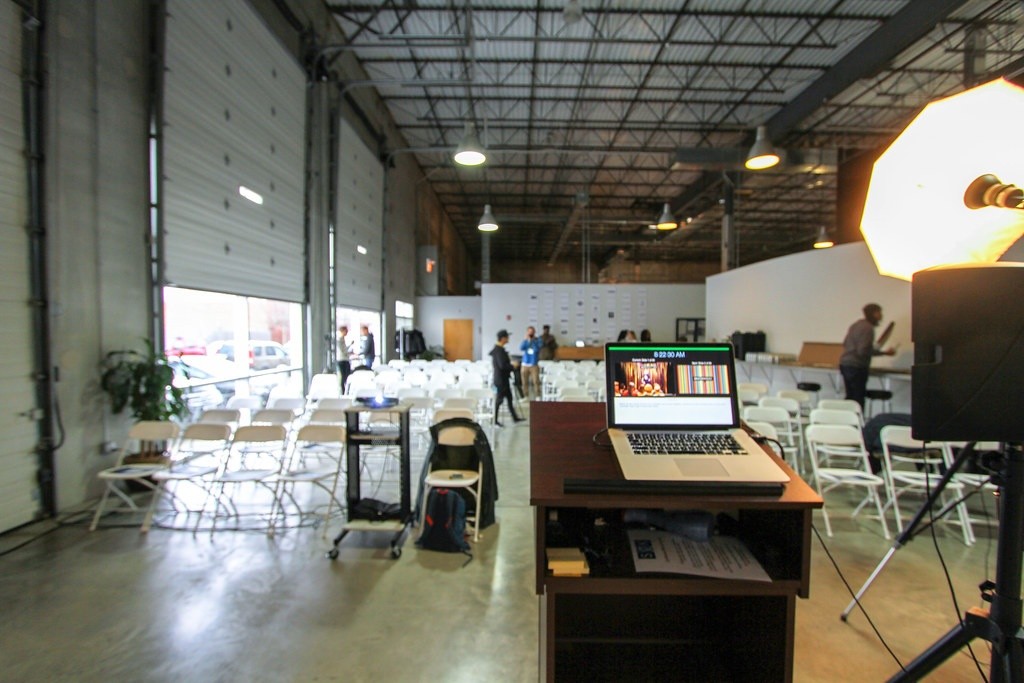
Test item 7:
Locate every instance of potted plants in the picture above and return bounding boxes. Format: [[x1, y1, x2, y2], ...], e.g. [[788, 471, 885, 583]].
[[97, 335, 211, 494]]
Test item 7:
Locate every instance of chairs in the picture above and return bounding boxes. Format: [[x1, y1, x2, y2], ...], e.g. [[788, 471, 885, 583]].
[[75, 358, 1003, 543]]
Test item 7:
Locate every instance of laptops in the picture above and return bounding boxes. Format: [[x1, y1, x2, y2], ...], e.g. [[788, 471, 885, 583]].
[[605, 343, 792, 486]]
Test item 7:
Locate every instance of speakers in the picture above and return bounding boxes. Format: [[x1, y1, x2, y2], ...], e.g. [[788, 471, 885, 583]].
[[911, 265, 1023, 446]]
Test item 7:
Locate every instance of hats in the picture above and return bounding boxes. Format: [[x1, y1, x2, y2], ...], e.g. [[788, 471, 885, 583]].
[[498, 330, 512, 340]]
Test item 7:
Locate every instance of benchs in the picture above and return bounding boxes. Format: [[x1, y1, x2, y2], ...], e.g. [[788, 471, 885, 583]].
[[781, 342, 843, 366]]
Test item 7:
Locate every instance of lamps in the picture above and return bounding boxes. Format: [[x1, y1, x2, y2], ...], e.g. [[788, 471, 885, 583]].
[[478, 202, 500, 232], [812, 224, 840, 250], [452, 113, 488, 167], [743, 125, 781, 171], [656, 200, 679, 231], [859, 74, 1024, 286]]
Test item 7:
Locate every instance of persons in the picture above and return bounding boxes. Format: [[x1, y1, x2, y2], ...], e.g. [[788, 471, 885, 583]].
[[336, 326, 354, 395], [357, 326, 375, 369], [612, 330, 652, 342], [838, 304, 895, 413], [518, 327, 541, 402], [614, 371, 665, 397], [490, 330, 527, 427], [538, 325, 558, 360]]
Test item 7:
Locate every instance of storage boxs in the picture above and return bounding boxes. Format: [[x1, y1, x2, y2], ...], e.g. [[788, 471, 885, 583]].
[[744, 351, 757, 361], [733, 331, 767, 360], [758, 351, 794, 363], [774, 355, 798, 364]]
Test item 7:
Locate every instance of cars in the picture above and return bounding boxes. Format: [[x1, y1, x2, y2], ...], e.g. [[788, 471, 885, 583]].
[[166, 338, 290, 409]]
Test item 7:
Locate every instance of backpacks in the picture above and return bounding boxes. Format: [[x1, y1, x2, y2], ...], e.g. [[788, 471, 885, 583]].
[[418, 490, 470, 554]]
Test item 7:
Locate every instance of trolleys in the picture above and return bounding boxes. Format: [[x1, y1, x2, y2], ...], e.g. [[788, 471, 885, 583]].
[[326, 402, 416, 561]]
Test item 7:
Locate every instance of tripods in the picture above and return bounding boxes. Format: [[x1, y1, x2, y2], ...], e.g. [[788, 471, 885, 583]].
[[885, 443, 1023, 682]]
